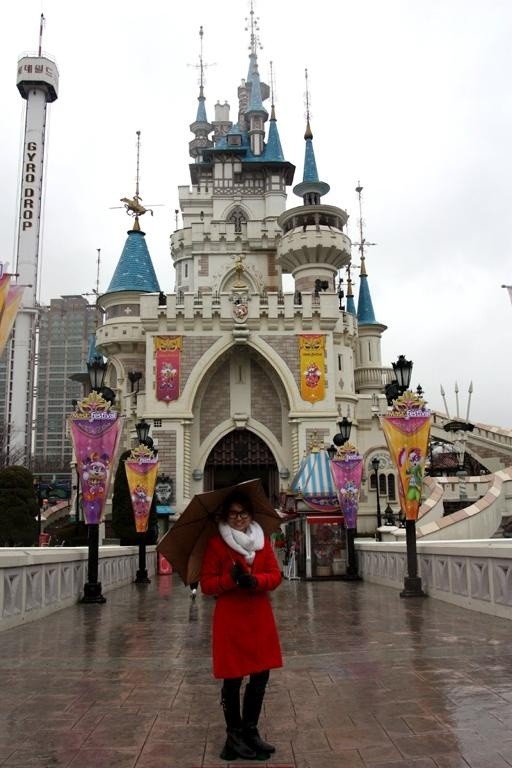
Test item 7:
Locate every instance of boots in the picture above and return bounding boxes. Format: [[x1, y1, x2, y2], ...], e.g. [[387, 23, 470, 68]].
[[241, 683, 277, 755], [220, 700, 258, 760]]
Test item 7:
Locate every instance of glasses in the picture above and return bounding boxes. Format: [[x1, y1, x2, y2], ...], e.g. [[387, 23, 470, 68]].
[[226, 510, 249, 519]]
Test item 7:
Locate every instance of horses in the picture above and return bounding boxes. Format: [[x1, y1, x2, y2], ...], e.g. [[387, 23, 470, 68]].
[[120, 197, 153, 218]]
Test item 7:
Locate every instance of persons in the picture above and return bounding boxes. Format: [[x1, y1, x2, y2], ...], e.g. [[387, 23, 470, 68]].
[[190, 581, 199, 600], [200, 488, 285, 762]]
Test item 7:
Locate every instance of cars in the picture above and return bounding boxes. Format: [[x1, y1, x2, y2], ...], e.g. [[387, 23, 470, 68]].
[[37, 481, 66, 489]]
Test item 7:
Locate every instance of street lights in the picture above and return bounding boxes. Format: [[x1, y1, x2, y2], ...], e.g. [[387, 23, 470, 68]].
[[370, 456, 383, 540], [132, 416, 152, 584], [390, 353, 428, 599], [76, 352, 110, 606], [326, 444, 338, 459], [336, 415, 364, 582], [382, 503, 406, 527]]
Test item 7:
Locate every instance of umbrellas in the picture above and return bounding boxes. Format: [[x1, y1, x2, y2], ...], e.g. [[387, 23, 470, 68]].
[[154, 476, 284, 588]]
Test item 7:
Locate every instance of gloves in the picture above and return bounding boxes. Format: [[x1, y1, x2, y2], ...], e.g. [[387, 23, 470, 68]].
[[231, 560, 258, 589]]
